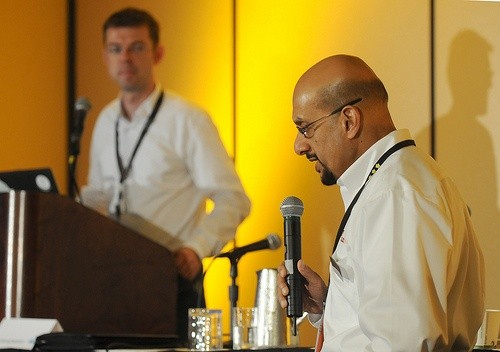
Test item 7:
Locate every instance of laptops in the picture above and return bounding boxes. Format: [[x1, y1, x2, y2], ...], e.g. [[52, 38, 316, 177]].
[[0, 168, 58, 193]]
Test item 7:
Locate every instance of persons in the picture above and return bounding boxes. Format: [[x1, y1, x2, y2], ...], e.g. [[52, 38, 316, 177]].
[[76, 8, 253, 348], [279, 54, 487, 352]]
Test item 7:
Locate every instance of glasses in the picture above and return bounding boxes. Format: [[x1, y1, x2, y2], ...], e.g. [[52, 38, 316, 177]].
[[296, 98, 363, 134]]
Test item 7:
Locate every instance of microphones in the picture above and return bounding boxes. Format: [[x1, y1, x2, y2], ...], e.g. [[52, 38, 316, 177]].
[[280, 196, 305, 318], [217, 233, 282, 257], [69, 98, 91, 169]]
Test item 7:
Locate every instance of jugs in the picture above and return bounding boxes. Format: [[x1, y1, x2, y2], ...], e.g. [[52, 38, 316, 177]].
[[248, 268, 308, 350]]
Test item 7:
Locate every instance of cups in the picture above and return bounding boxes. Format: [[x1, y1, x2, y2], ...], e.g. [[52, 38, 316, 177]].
[[207, 310, 223, 349], [188, 308, 211, 349], [232, 308, 257, 349]]
